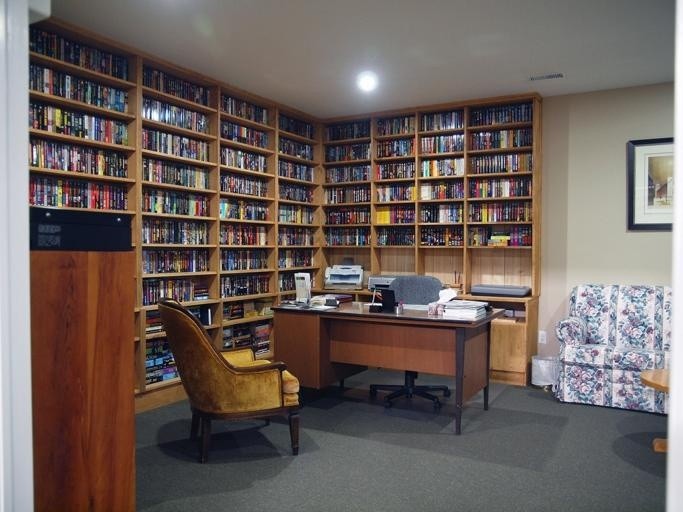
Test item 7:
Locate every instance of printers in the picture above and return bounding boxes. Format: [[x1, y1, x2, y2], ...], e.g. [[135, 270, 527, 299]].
[[325, 265, 363, 289]]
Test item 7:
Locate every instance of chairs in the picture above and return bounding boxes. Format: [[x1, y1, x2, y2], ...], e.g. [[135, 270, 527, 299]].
[[157, 297, 299, 464]]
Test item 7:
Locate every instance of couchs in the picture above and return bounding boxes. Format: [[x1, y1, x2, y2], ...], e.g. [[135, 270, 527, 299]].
[[554, 283, 671, 415]]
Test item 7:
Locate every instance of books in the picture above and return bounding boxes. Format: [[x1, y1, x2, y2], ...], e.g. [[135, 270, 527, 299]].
[[141, 67, 315, 160], [144, 307, 212, 385], [441, 298, 490, 322], [29, 23, 131, 212], [222, 296, 272, 357], [323, 293, 352, 300], [278, 226, 315, 292], [502, 307, 525, 318], [337, 297, 353, 303], [140, 147, 314, 224], [142, 218, 213, 305], [322, 102, 535, 248], [218, 223, 270, 298]]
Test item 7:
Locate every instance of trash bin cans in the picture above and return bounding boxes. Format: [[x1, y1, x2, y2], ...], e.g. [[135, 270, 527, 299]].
[[531, 355, 558, 385]]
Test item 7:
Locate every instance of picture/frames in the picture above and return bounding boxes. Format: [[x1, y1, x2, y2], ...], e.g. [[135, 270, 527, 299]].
[[625, 137, 673, 231]]
